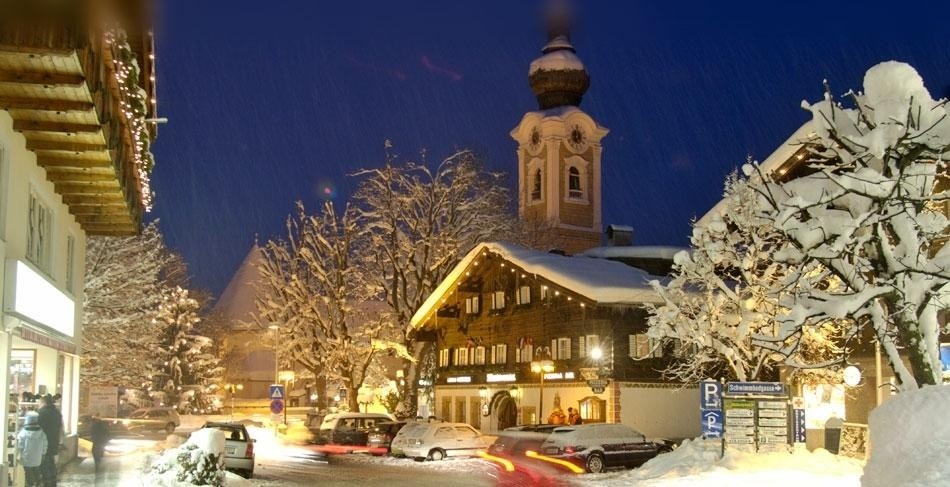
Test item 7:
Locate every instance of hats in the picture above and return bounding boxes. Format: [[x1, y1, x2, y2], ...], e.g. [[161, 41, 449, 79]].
[[24, 410, 40, 424]]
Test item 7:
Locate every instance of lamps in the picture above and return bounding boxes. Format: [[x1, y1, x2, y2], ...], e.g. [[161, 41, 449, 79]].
[[510, 385, 520, 398], [479, 386, 487, 397], [482, 401, 490, 415]]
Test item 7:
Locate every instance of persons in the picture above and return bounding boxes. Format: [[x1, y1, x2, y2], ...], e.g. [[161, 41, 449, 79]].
[[567, 406, 576, 424], [16, 410, 49, 486], [37, 393, 61, 487], [572, 408, 582, 425]]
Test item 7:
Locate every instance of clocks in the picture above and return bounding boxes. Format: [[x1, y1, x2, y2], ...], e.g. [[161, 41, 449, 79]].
[[562, 118, 591, 154], [524, 123, 545, 156]]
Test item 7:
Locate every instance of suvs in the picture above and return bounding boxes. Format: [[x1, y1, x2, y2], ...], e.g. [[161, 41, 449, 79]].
[[114, 408, 184, 433], [196, 421, 256, 476]]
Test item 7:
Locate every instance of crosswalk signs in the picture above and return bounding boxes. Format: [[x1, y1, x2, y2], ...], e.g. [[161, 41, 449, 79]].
[[270, 385, 283, 399]]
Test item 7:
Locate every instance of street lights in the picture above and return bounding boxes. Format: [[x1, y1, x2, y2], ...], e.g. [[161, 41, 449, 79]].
[[265, 319, 285, 437], [224, 383, 245, 422], [530, 359, 557, 423]]
